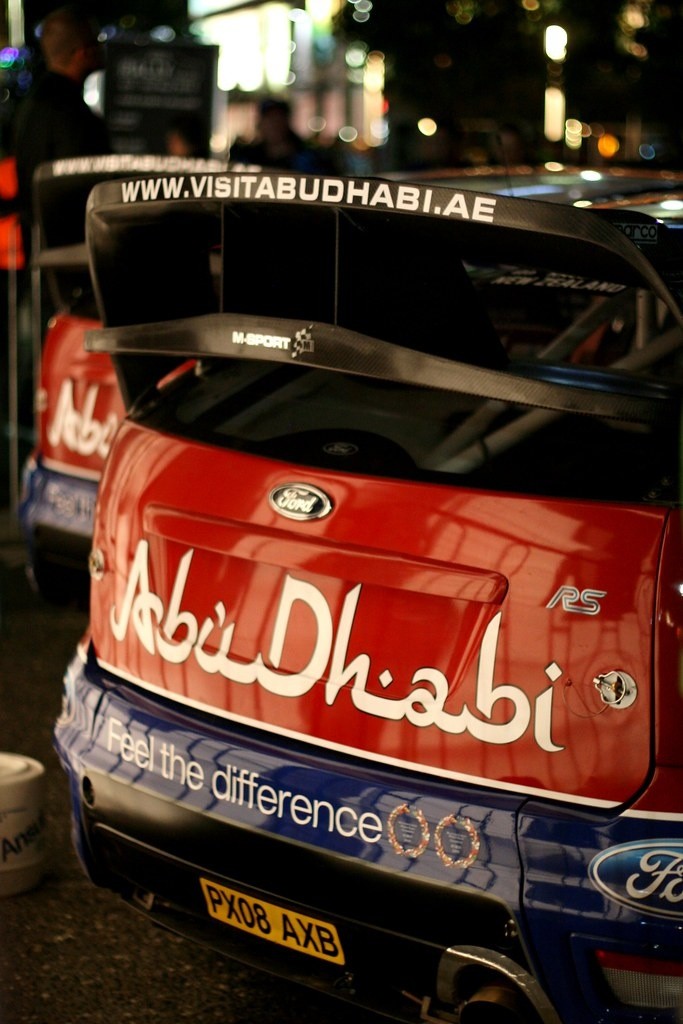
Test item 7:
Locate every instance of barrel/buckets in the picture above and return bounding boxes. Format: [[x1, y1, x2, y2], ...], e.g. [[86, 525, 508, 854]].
[[0, 750, 47, 898]]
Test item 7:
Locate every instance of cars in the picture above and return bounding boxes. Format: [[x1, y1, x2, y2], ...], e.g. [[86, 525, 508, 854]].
[[15, 155, 683, 1024]]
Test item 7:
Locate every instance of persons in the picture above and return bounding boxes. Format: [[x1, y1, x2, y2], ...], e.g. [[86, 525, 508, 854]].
[[229, 95, 526, 175], [10, 10, 119, 340]]
